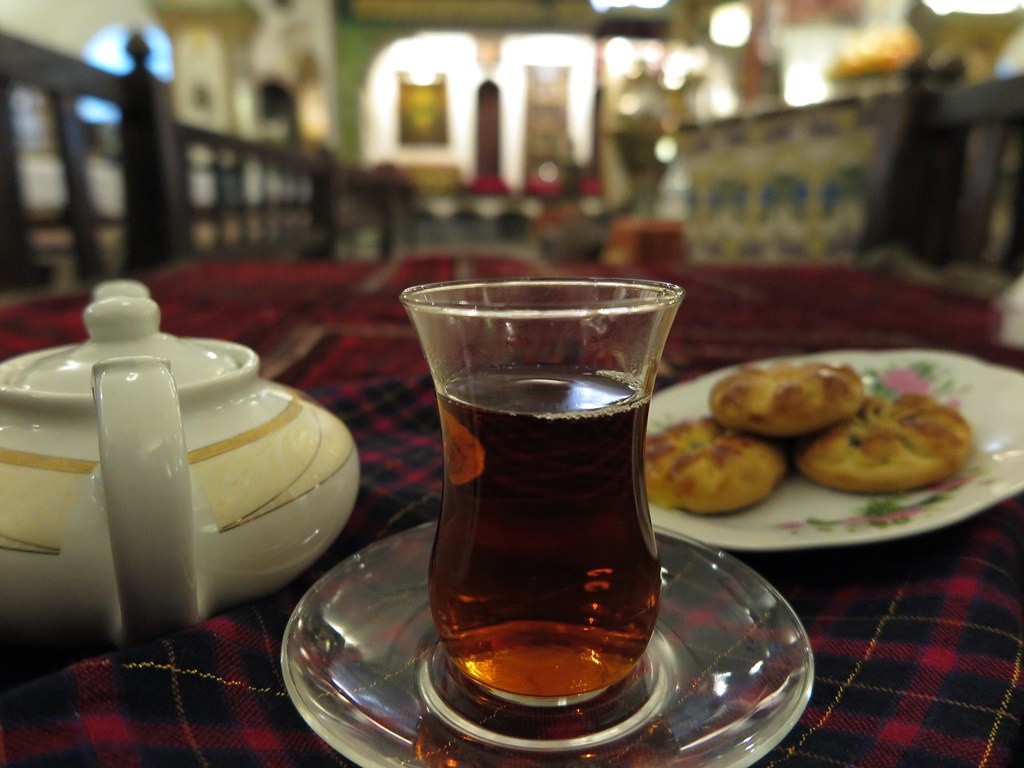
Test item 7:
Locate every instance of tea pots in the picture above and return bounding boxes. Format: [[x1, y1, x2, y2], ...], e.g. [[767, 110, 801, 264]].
[[0, 279, 361, 647]]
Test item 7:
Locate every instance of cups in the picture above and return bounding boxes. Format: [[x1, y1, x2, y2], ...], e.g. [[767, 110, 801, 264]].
[[399, 276, 689, 706]]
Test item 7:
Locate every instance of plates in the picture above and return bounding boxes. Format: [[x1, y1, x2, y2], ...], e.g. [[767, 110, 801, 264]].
[[643, 348, 1024, 550], [281, 517, 814, 768]]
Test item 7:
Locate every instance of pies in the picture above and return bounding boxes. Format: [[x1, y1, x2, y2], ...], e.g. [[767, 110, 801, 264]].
[[643, 364, 977, 513]]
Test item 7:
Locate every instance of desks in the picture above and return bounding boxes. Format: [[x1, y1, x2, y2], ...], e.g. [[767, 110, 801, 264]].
[[0, 257, 1024, 768]]
